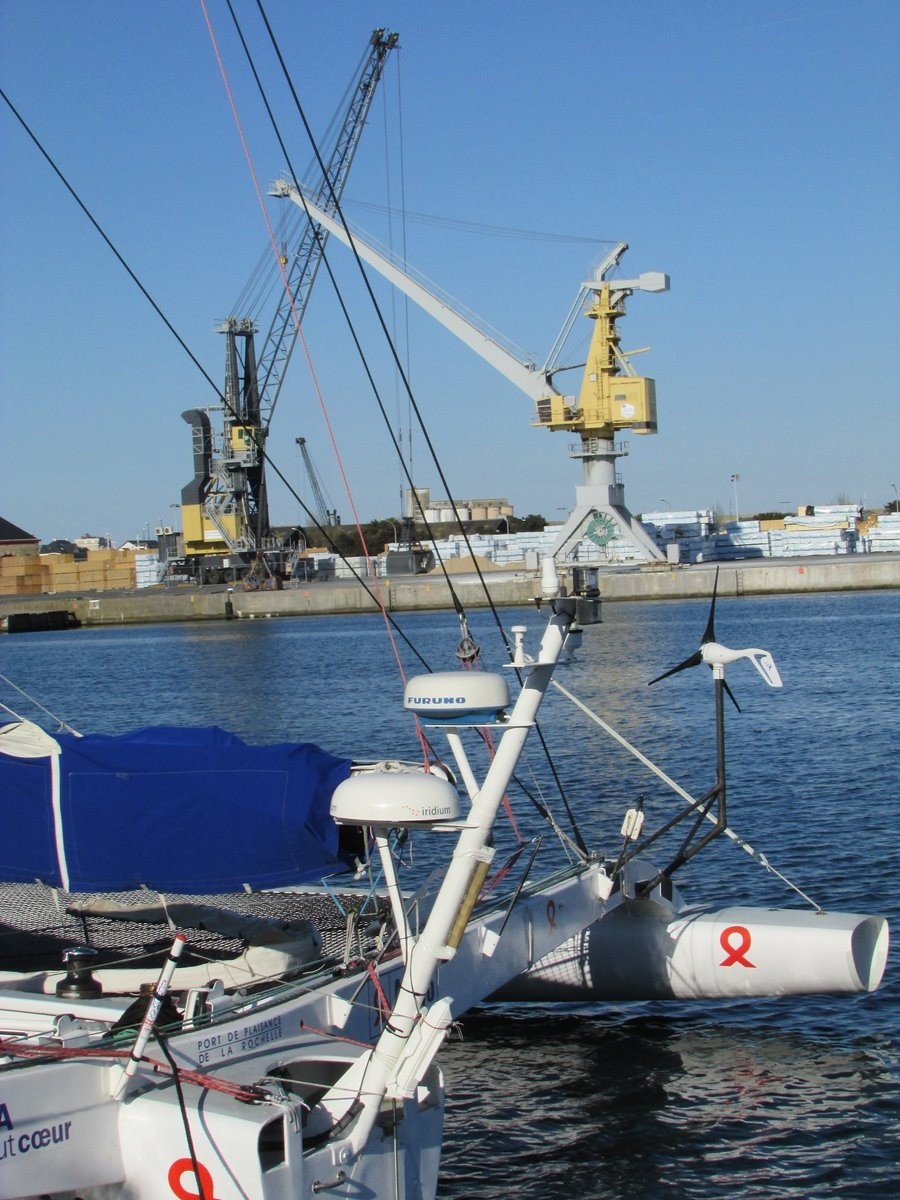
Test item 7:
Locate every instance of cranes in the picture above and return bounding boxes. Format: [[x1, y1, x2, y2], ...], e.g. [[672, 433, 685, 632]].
[[295, 437, 331, 525], [266, 176, 677, 564], [178, 26, 402, 557]]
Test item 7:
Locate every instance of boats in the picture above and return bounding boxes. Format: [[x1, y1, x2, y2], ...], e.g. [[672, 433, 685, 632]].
[[1, 555, 894, 1200]]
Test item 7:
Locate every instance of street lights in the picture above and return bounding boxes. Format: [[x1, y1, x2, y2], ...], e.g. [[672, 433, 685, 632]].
[[731, 473, 740, 522]]
